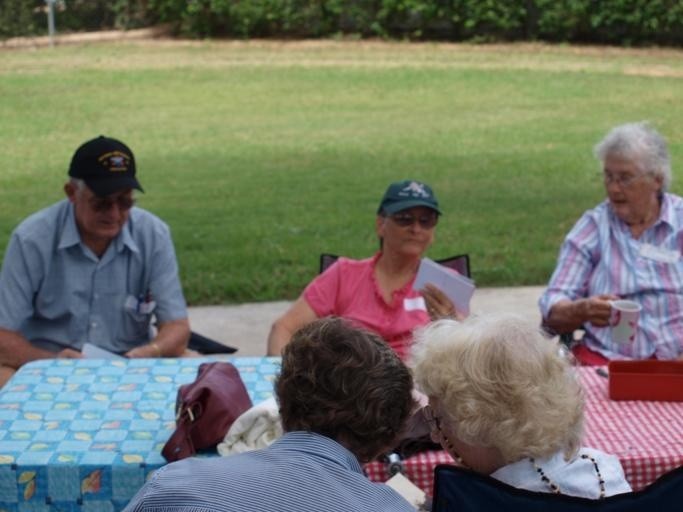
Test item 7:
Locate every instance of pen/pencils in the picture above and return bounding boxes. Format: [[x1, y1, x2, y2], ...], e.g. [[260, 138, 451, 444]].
[[139, 288, 150, 303]]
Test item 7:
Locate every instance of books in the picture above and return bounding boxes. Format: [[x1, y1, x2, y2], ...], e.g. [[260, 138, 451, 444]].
[[412, 257, 477, 314], [383, 469, 435, 511]]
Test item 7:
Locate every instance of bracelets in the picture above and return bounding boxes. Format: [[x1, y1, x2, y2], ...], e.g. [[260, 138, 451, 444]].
[[149, 341, 161, 356]]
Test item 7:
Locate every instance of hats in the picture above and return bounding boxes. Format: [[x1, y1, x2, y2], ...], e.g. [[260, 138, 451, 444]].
[[377, 179, 444, 217], [68, 135, 145, 196]]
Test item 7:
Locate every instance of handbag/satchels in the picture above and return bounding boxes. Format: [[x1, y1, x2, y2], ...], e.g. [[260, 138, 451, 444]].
[[161, 362, 253, 464]]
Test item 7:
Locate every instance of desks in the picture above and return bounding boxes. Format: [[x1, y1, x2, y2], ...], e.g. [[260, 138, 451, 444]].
[[2, 352, 683, 509]]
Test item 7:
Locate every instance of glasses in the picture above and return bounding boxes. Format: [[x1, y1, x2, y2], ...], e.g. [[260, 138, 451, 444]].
[[84, 196, 136, 213], [388, 210, 437, 229], [597, 166, 657, 187], [422, 405, 445, 435]]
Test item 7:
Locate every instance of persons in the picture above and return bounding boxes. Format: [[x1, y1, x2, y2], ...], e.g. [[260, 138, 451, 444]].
[[113, 312, 418, 512], [265, 177, 469, 360], [405, 311, 632, 512], [535, 120, 681, 367], [0, 132, 202, 393]]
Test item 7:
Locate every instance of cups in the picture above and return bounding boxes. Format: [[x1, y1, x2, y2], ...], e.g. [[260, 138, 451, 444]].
[[605, 299, 643, 345]]
